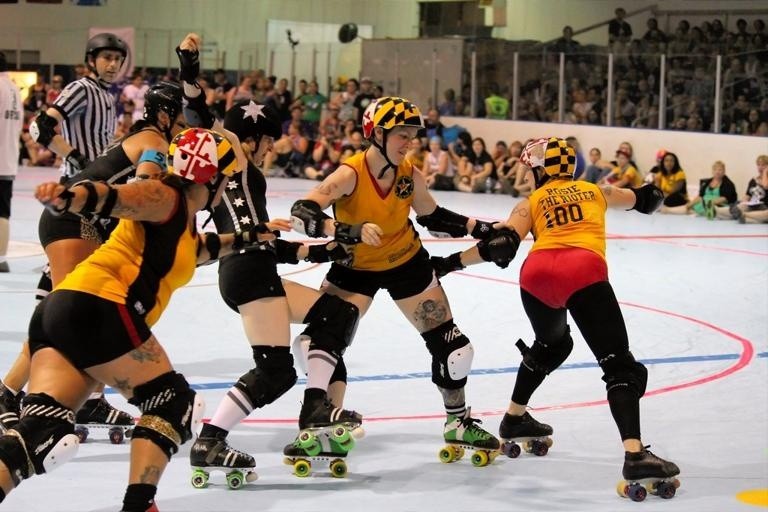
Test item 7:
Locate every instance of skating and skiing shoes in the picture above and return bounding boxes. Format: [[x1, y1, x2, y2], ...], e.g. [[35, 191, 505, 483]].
[[439, 416, 501, 466], [190, 433, 257, 489], [284, 440, 347, 477], [76, 397, 136, 444], [297, 395, 363, 456], [617, 448, 680, 502], [499, 410, 554, 458]]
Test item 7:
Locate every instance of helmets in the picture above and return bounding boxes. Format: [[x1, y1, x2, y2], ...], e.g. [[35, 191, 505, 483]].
[[143, 81, 282, 186], [520, 137, 577, 179], [85, 33, 128, 69], [363, 97, 425, 138]]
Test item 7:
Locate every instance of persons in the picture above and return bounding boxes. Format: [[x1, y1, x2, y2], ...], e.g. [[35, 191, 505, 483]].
[[27, 30, 130, 303], [0, 78, 184, 446], [702, 155, 768, 223], [684, 161, 737, 219], [0, 51, 23, 272], [279, 95, 504, 479], [19, 64, 531, 196], [529, 9, 768, 138], [566, 137, 690, 208], [1, 124, 294, 512], [430, 137, 683, 482], [175, 30, 364, 490]]
